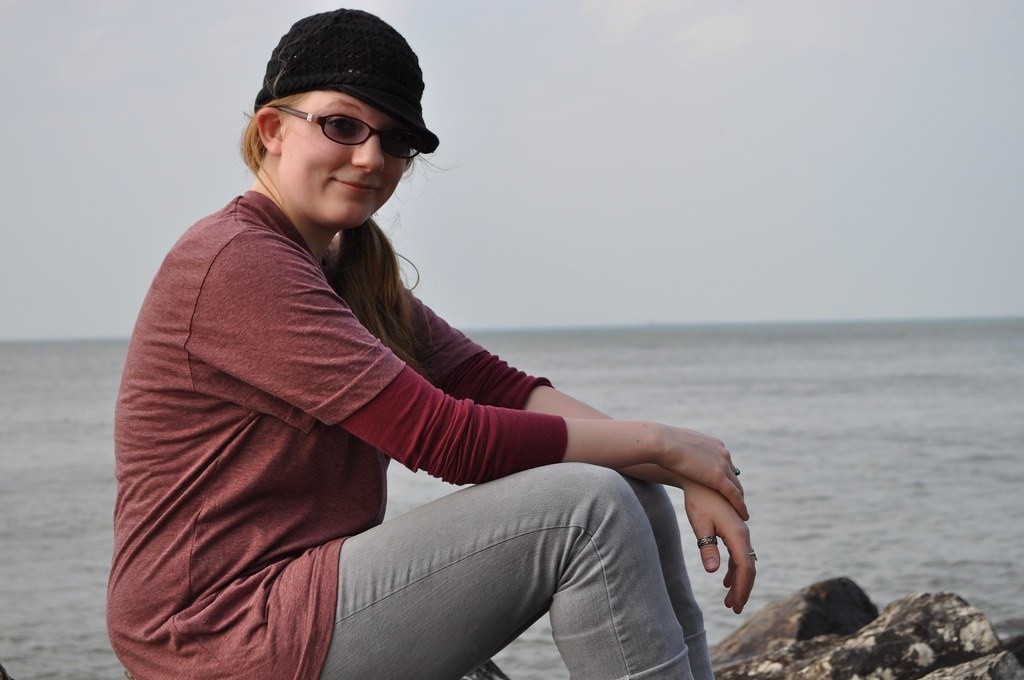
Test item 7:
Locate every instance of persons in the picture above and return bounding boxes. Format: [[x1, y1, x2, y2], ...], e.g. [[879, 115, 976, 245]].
[[107, 9, 760, 680]]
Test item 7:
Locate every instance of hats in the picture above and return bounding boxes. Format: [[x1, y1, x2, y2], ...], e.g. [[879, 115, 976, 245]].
[[254, 9, 439, 153]]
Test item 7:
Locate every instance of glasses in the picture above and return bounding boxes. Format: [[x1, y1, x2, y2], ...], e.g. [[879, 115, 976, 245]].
[[271, 105, 429, 159]]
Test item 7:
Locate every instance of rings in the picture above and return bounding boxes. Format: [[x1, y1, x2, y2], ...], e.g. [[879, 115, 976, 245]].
[[697, 535, 718, 547], [733, 467, 741, 477], [749, 548, 757, 562]]
